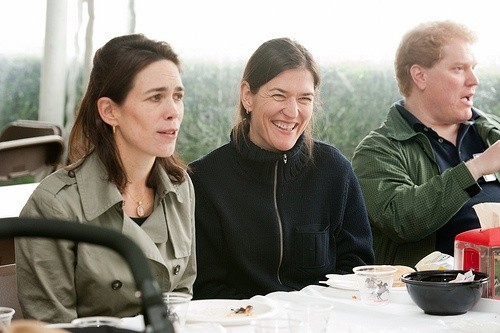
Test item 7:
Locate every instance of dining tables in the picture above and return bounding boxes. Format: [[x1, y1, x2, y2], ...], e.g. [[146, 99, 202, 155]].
[[44, 286, 500, 333]]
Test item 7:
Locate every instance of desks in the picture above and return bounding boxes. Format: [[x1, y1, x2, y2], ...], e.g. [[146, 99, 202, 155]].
[[0, 182, 41, 266]]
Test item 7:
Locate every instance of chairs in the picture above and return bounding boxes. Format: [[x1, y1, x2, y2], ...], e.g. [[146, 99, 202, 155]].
[[0, 118, 66, 184]]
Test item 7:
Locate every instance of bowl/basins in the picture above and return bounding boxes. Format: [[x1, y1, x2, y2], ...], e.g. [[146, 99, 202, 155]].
[[400, 269, 491, 316]]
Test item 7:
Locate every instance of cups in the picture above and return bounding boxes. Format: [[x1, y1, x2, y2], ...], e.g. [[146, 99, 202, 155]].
[[161, 292, 193, 333], [71, 316, 119, 328], [287, 301, 335, 333], [352, 265, 398, 305], [0, 307, 15, 329], [251, 317, 294, 333]]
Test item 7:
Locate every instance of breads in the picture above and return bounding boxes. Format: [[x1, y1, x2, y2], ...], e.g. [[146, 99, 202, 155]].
[[371, 265, 416, 287]]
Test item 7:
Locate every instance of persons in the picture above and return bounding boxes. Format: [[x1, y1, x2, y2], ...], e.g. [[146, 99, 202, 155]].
[[350, 19, 500, 271], [14, 33, 197, 323], [186, 37, 376, 300]]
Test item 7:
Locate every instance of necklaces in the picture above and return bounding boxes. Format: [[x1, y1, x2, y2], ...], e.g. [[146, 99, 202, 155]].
[[127, 191, 148, 217]]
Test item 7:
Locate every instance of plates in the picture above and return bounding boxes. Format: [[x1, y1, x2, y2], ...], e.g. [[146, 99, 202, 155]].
[[186, 300, 276, 326], [326, 274, 359, 290]]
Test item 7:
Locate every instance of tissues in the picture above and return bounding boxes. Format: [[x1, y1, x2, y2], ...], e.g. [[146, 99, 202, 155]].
[[454, 203, 500, 300]]
